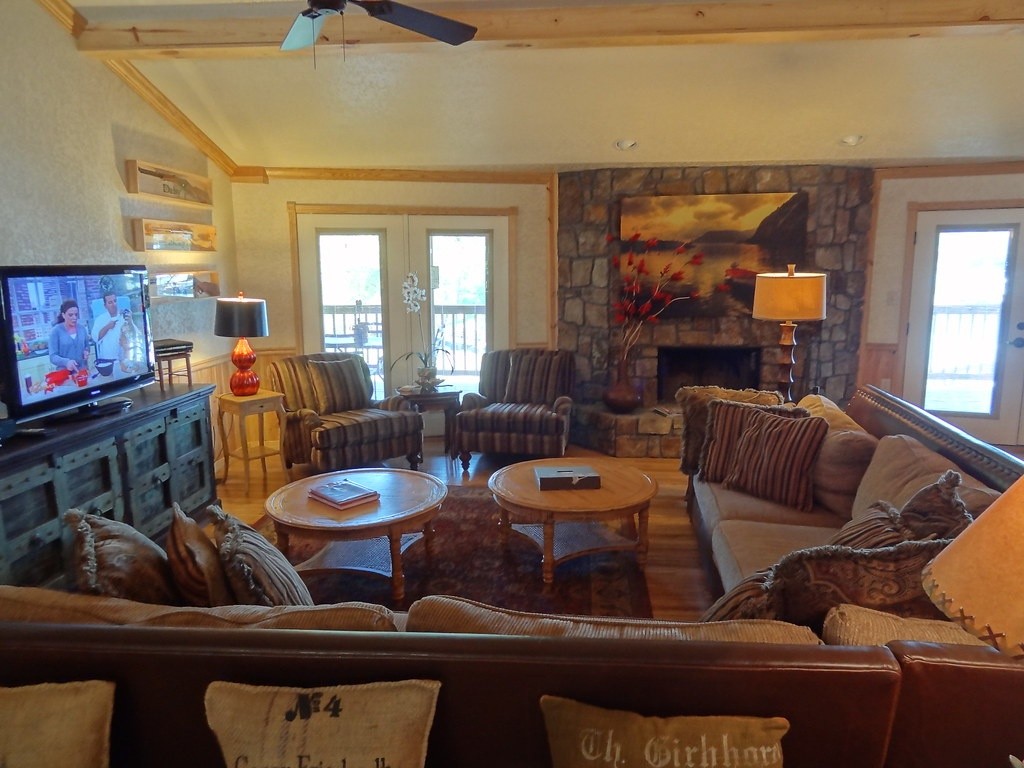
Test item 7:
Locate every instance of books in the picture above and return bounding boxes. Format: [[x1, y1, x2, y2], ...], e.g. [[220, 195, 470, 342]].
[[308, 478, 380, 510]]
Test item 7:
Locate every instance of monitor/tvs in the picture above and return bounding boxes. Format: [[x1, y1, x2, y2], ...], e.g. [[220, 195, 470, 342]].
[[0, 265, 156, 426]]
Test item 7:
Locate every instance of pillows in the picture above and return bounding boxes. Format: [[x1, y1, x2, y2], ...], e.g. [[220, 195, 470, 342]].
[[1, 385, 1007, 768]]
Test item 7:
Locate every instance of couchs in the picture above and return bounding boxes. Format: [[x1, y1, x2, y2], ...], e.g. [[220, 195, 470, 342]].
[[452, 347, 574, 469], [0, 621, 899, 768], [686, 384, 1024, 767], [270, 351, 424, 479]]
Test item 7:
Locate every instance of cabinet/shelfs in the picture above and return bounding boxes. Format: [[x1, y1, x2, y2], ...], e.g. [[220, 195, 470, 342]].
[[124, 158, 220, 303], [0, 383, 224, 587]]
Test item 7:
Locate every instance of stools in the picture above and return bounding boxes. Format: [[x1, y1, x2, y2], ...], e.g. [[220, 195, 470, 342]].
[[155, 354, 192, 389]]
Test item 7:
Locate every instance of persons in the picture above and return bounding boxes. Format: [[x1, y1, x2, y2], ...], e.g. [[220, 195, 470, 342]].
[[91, 292, 138, 359], [47, 299, 90, 375]]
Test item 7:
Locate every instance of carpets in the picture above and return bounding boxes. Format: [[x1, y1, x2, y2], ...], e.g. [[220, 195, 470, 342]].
[[251, 482, 654, 618]]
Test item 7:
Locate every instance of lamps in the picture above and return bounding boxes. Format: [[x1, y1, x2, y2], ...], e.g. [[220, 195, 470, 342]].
[[753, 263, 828, 403], [212, 291, 270, 396], [921, 475, 1024, 659]]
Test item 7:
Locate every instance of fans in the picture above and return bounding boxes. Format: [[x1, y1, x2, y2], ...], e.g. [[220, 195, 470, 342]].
[[281, 0, 476, 52]]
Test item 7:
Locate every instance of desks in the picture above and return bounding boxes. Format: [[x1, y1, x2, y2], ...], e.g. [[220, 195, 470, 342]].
[[487, 458, 658, 585], [263, 469, 450, 608], [215, 388, 285, 495], [393, 386, 463, 453]]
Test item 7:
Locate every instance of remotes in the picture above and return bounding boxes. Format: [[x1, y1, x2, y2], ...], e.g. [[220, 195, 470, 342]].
[[15, 428, 57, 437]]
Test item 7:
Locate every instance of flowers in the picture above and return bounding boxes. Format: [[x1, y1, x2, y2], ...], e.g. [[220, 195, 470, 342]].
[[602, 231, 759, 363], [388, 273, 450, 371]]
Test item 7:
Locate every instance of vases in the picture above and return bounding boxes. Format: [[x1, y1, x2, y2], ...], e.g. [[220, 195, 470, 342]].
[[604, 361, 643, 414], [414, 368, 444, 393]]
